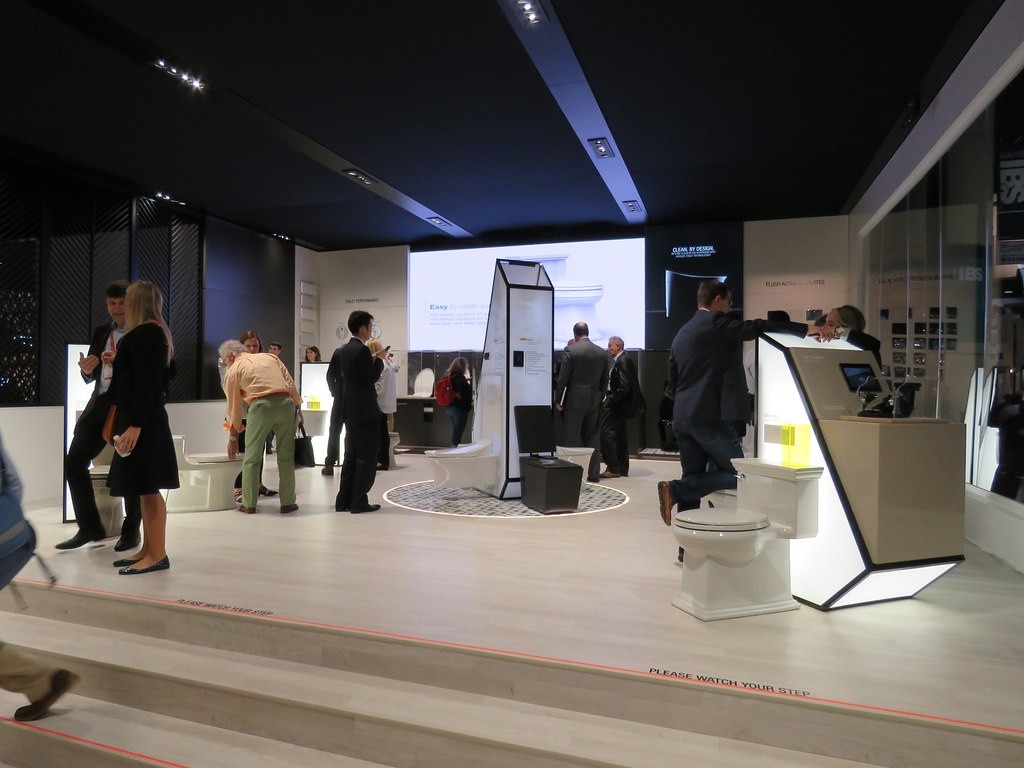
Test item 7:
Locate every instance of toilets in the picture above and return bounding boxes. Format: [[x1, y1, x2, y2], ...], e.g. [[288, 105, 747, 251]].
[[378, 431, 401, 470], [552, 445, 596, 493], [424, 382, 503, 490], [75, 409, 126, 540], [403, 367, 436, 398], [512, 404, 584, 515], [668, 455, 825, 624], [158, 434, 246, 514]]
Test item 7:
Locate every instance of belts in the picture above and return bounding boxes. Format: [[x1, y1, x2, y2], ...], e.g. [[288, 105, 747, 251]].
[[607, 391, 613, 394]]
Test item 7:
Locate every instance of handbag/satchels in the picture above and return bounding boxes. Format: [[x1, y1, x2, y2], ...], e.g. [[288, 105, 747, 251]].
[[294, 422, 315, 471]]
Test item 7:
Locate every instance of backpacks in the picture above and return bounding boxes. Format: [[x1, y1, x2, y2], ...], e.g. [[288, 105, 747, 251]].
[[435, 372, 461, 407]]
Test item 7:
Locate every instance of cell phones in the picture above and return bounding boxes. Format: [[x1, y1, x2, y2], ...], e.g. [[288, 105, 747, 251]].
[[385, 346, 391, 351]]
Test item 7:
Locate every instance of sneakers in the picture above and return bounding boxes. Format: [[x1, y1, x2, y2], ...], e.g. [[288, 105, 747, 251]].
[[678, 546, 684, 562], [658, 481, 673, 526]]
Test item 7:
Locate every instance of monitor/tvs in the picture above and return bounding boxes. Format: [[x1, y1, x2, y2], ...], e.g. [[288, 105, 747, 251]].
[[840, 363, 882, 392]]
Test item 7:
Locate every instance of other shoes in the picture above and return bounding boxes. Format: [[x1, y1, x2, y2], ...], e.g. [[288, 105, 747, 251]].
[[119, 555, 170, 575], [280, 504, 299, 513], [599, 471, 628, 478], [113, 559, 143, 567], [239, 505, 256, 514], [376, 464, 389, 471], [587, 476, 599, 482], [322, 466, 334, 476], [14, 669, 81, 720]]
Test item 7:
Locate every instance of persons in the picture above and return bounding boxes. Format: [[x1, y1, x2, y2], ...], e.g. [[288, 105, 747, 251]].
[[657, 277, 833, 526], [53, 278, 180, 576], [0, 442, 81, 721], [217, 309, 401, 515], [814, 304, 882, 375], [446, 358, 473, 448], [553, 322, 644, 482]]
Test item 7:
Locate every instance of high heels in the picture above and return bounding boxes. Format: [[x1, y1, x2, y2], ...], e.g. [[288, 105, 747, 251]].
[[234, 491, 242, 503], [259, 486, 278, 497]]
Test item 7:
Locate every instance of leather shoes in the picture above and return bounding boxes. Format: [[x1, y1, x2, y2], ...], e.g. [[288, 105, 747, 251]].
[[114, 533, 141, 552], [55, 526, 106, 549], [336, 504, 381, 514]]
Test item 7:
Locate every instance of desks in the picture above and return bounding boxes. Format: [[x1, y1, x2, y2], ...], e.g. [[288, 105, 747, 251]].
[[393, 397, 474, 451]]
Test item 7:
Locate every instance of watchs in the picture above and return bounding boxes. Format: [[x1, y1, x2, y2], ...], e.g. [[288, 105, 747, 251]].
[[834, 325, 845, 338]]
